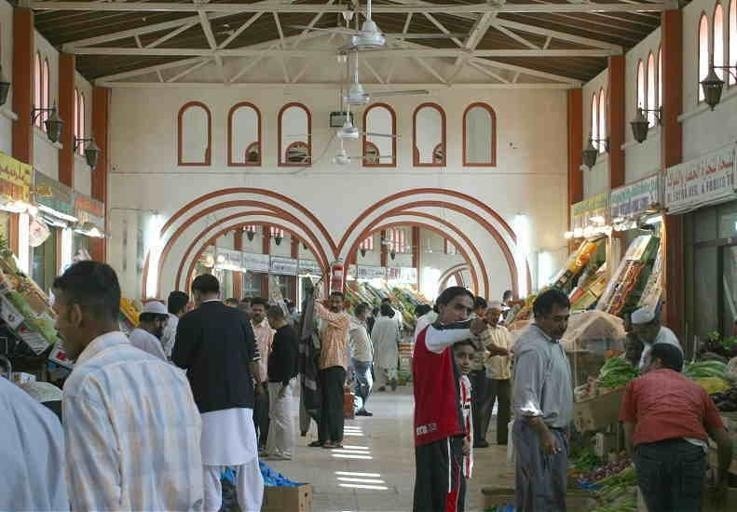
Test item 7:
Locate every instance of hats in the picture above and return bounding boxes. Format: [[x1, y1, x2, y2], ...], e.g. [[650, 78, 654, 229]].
[[138, 300, 171, 320], [631, 305, 656, 325], [488, 299, 504, 311]]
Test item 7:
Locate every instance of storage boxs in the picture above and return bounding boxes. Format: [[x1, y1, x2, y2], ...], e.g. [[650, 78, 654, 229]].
[[399, 355, 412, 371], [569, 289, 597, 311], [234, 482, 313, 512], [11, 372, 36, 385], [575, 389, 625, 431], [479, 487, 517, 512], [583, 271, 608, 298], [566, 490, 592, 512], [0, 255, 80, 369], [399, 342, 415, 358]]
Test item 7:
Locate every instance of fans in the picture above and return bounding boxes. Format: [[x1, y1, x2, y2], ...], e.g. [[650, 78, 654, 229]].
[[279, 0, 429, 166]]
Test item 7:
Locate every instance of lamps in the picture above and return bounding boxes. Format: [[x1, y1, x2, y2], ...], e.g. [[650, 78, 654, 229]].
[[359, 242, 366, 257], [697, 63, 736, 111], [389, 246, 396, 260], [268, 232, 283, 246], [0, 64, 11, 105], [629, 106, 663, 143], [73, 135, 100, 169], [581, 134, 609, 171], [31, 97, 66, 143], [243, 227, 256, 241]]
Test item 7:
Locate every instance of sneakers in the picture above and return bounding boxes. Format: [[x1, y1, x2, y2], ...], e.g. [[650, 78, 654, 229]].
[[306, 439, 342, 449], [356, 408, 374, 416]]
